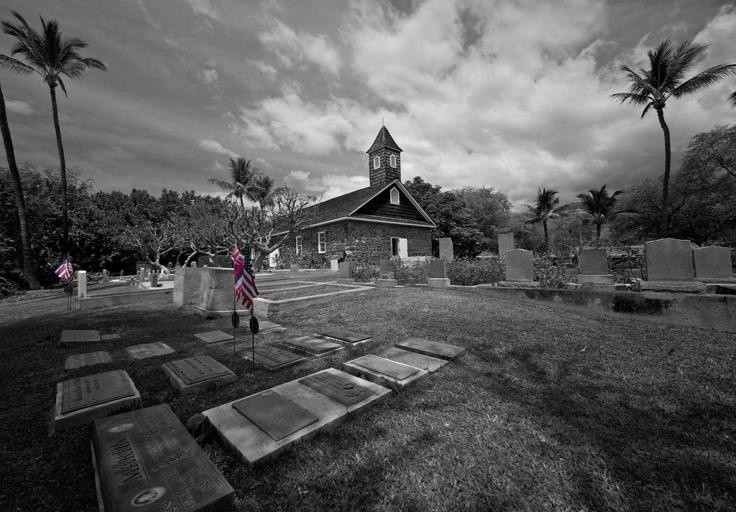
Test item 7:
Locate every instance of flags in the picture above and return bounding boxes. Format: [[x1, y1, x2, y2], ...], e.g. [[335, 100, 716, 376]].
[[232, 241, 259, 314], [54, 257, 74, 283]]
